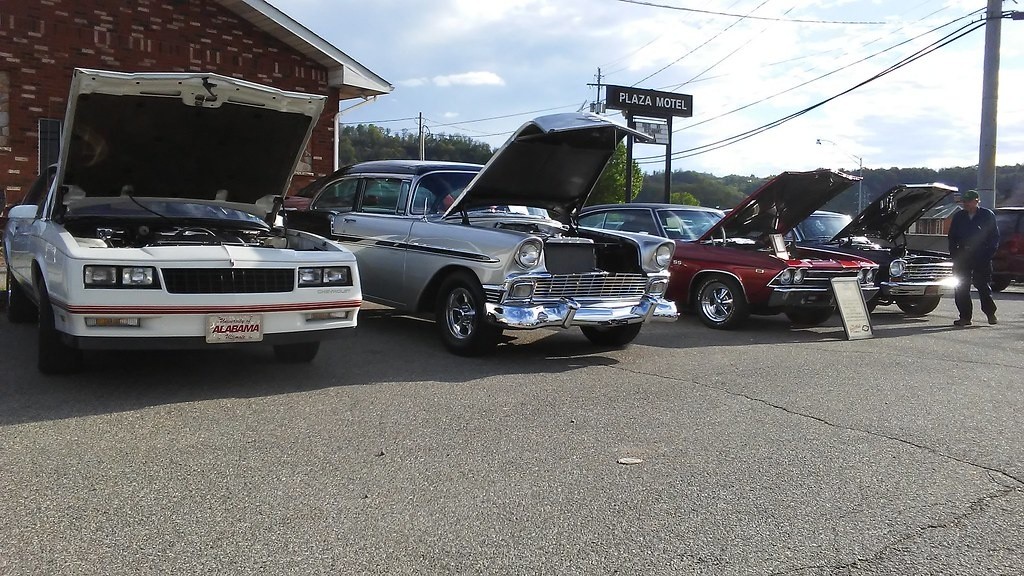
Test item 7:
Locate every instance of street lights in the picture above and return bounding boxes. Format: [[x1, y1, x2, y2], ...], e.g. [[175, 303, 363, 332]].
[[813, 137, 864, 219]]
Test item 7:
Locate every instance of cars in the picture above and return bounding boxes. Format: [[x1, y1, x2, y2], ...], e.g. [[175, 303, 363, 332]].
[[702, 181, 963, 317], [277, 110, 683, 353], [975, 205, 1024, 294], [568, 167, 880, 328], [1, 67, 362, 376]]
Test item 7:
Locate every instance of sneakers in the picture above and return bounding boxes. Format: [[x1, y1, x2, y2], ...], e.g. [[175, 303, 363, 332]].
[[954, 318, 971, 325], [988, 314, 997, 324]]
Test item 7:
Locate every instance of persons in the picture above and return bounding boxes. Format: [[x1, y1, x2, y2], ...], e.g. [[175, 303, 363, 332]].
[[947, 190, 1000, 325]]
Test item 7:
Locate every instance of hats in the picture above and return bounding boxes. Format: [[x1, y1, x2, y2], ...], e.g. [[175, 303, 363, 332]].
[[961, 190, 978, 201]]
